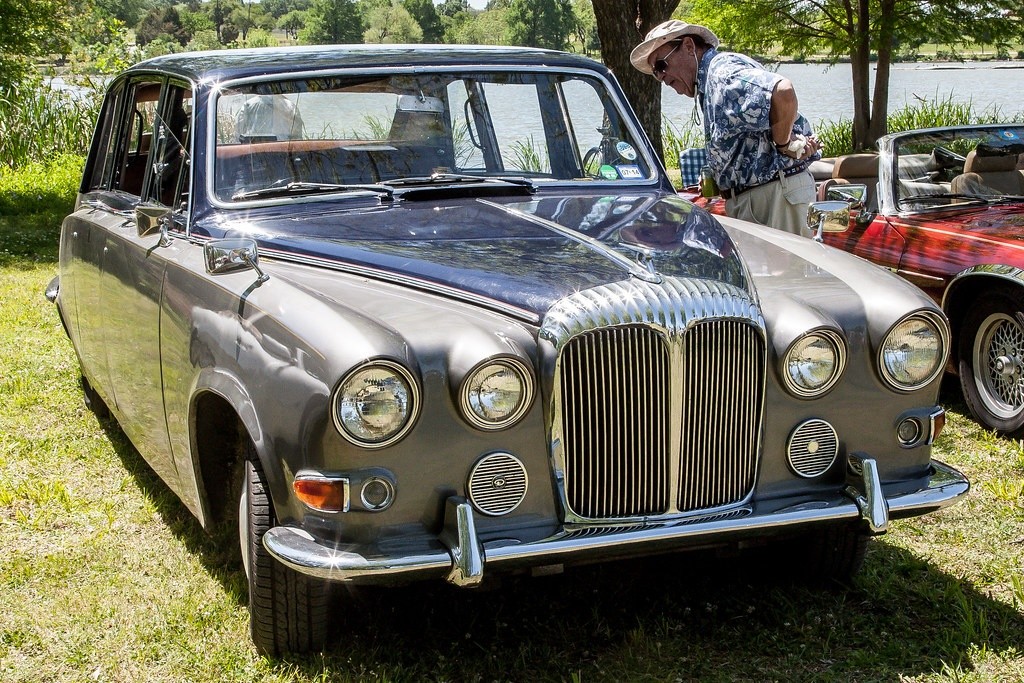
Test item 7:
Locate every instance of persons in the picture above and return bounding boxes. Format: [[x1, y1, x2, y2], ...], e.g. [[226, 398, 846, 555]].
[[233, 88, 308, 143], [628, 19, 824, 239]]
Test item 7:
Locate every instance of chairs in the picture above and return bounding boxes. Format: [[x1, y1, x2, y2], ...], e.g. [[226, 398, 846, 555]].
[[818, 154, 914, 213], [951, 149, 1024, 203]]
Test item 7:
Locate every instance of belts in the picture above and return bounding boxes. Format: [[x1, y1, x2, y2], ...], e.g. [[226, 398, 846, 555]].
[[721, 161, 805, 199]]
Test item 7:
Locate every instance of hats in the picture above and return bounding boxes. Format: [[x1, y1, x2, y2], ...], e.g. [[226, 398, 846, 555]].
[[630, 19, 719, 75]]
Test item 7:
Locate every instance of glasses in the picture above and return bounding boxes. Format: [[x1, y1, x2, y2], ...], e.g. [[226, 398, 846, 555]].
[[652, 45, 679, 83]]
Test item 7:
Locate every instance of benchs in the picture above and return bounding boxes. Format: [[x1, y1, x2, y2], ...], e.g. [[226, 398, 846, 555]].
[[180, 141, 451, 198], [815, 180, 952, 208]]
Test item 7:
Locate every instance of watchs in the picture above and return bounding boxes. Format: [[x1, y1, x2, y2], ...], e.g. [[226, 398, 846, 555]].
[[772, 139, 791, 150]]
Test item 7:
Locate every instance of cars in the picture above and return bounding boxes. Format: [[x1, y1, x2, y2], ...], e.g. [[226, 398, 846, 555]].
[[674, 122, 1023, 432], [43, 45, 969, 659]]
